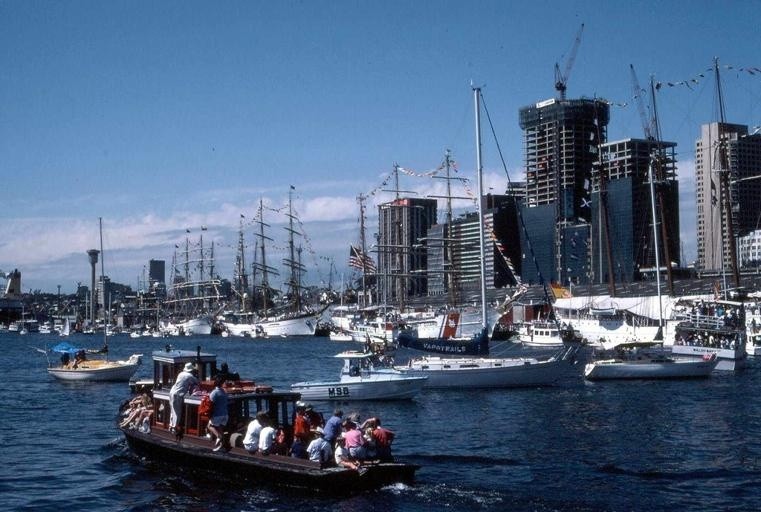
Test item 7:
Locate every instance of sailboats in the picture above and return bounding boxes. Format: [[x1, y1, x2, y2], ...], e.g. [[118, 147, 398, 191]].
[[158, 146, 504, 351]]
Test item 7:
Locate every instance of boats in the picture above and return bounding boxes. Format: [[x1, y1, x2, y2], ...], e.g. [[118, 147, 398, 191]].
[[115, 344, 423, 498], [392, 354, 569, 390], [584, 358, 721, 381], [46, 353, 144, 383], [507, 294, 761, 361], [289, 349, 429, 402]]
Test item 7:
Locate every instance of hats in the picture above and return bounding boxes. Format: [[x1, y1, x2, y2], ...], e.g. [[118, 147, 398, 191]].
[[183, 362, 196, 372], [315, 426, 327, 436]]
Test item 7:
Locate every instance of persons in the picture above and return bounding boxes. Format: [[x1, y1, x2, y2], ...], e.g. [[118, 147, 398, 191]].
[[59, 349, 86, 368], [675, 297, 761, 354], [121, 361, 396, 479], [374, 344, 387, 364]]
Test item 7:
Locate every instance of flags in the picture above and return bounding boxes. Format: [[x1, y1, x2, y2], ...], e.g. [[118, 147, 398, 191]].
[[548, 282, 574, 302], [348, 244, 378, 276], [714, 280, 721, 301]]
